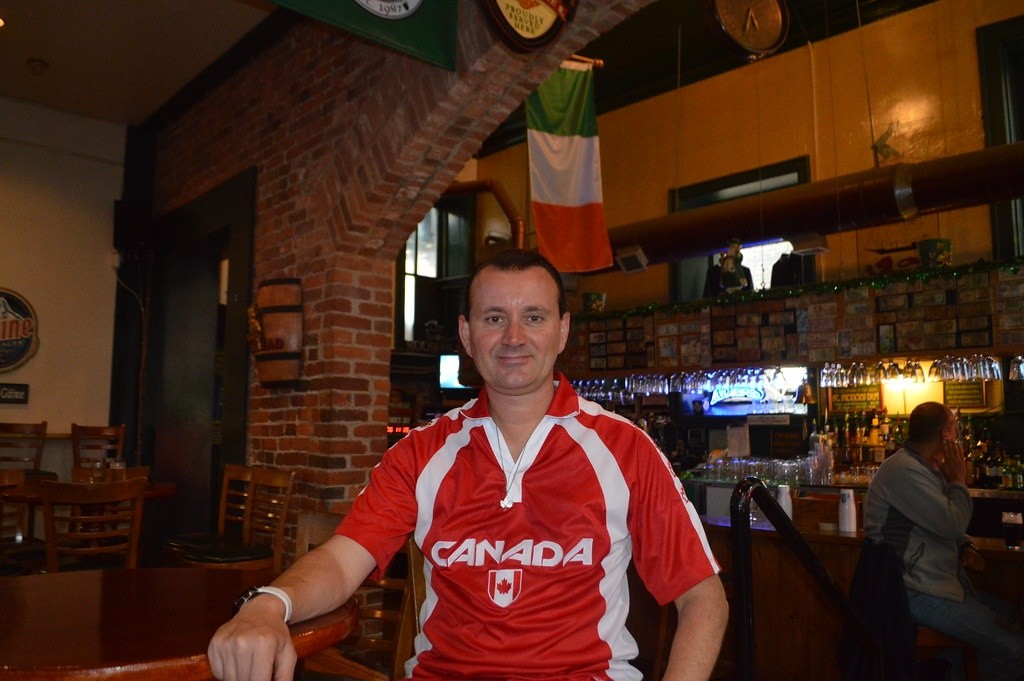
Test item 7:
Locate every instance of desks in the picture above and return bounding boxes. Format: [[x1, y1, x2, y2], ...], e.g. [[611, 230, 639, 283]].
[[0, 566, 355, 681], [0, 466, 179, 563]]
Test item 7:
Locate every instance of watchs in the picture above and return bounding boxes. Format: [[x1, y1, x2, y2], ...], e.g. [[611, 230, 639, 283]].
[[231, 586, 292, 622]]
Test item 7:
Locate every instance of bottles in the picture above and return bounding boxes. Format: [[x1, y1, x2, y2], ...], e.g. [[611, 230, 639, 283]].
[[648, 412, 654, 427], [619, 371, 669, 405], [928, 353, 1001, 382], [806, 405, 910, 472], [637, 417, 647, 434], [953, 403, 1024, 489], [571, 377, 620, 400], [1009, 355, 1024, 381], [819, 359, 925, 388]]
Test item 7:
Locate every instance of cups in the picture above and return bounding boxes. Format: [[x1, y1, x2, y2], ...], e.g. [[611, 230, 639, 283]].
[[813, 468, 834, 487], [703, 456, 812, 483], [670, 365, 784, 395], [838, 465, 880, 486], [839, 488, 856, 533], [1001, 512, 1023, 549], [91, 457, 106, 483], [109, 460, 126, 481], [776, 484, 793, 521]]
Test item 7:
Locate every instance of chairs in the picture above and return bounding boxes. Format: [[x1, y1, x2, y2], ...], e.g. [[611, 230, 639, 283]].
[[71, 423, 128, 533], [0, 420, 57, 537], [40, 476, 150, 574], [290, 509, 424, 681], [181, 464, 298, 568], [161, 464, 255, 564]]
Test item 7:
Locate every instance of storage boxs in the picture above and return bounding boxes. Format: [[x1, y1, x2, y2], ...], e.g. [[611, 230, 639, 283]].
[[585, 257, 1024, 378]]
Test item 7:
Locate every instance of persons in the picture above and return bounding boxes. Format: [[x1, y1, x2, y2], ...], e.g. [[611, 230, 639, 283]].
[[208, 249, 729, 681], [862, 402, 1024, 681]]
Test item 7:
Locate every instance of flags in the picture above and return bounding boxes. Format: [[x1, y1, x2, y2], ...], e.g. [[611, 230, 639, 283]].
[[526, 60, 614, 272]]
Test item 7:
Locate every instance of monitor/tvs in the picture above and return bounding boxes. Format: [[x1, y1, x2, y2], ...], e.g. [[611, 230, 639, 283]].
[[439, 354, 478, 391]]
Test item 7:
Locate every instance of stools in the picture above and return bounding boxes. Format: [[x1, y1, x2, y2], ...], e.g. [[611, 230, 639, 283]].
[[917, 624, 981, 681]]
